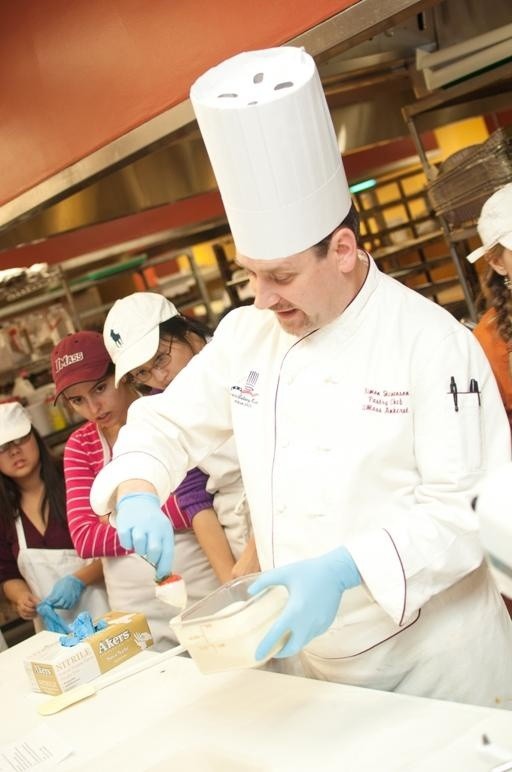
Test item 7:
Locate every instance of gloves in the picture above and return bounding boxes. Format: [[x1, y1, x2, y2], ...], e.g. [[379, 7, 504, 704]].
[[246, 546, 363, 660], [115, 493, 175, 581], [33, 574, 108, 646]]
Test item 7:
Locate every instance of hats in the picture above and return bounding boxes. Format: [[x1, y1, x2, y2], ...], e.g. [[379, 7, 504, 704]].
[[189, 47, 352, 261], [0, 401, 34, 446], [466, 181, 512, 263], [104, 291, 180, 389], [50, 331, 111, 406]]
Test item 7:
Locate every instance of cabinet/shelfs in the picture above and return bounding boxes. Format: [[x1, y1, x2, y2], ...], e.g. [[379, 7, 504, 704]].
[[0, 60, 512, 446]]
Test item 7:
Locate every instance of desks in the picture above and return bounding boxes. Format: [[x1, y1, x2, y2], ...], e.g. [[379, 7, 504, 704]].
[[0, 628, 512, 772]]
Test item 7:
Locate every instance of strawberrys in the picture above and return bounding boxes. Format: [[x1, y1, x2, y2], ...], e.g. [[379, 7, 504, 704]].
[[155, 575, 182, 587]]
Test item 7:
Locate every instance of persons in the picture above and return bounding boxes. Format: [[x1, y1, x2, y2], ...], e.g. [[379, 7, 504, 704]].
[[1, 396, 113, 638], [45, 330, 227, 622], [467, 183, 512, 440], [86, 199, 512, 690], [103, 286, 267, 582]]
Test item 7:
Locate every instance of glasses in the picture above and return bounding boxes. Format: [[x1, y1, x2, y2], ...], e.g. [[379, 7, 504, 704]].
[[134, 337, 173, 382]]
